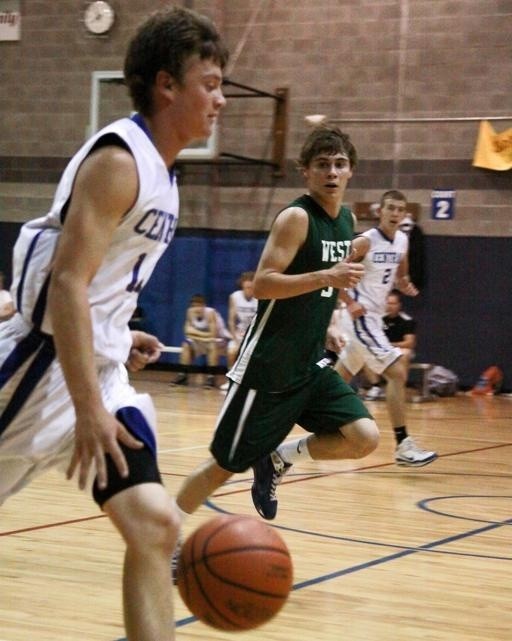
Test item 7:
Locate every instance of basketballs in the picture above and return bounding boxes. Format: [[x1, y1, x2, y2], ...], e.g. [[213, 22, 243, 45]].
[[176, 515, 293, 631]]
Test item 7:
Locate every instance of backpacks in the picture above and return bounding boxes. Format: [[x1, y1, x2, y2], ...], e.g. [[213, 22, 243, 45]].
[[472, 366, 504, 396]]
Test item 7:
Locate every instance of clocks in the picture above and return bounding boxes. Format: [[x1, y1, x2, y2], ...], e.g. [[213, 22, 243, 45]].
[[84, 1, 116, 34]]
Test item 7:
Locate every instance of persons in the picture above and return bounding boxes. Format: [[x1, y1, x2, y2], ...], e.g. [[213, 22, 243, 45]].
[[333, 189, 437, 467], [169, 128, 381, 578], [169, 271, 414, 401], [1, 8, 228, 640], [0, 272, 15, 329]]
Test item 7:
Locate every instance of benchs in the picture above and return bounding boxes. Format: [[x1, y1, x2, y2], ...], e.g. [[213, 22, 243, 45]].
[[160, 344, 436, 402]]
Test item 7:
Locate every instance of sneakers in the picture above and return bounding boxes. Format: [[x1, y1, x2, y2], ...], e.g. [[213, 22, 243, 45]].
[[202, 376, 216, 389], [364, 384, 383, 401], [249, 445, 293, 522], [394, 435, 438, 467], [219, 379, 231, 390], [170, 533, 183, 586], [168, 373, 188, 387]]
